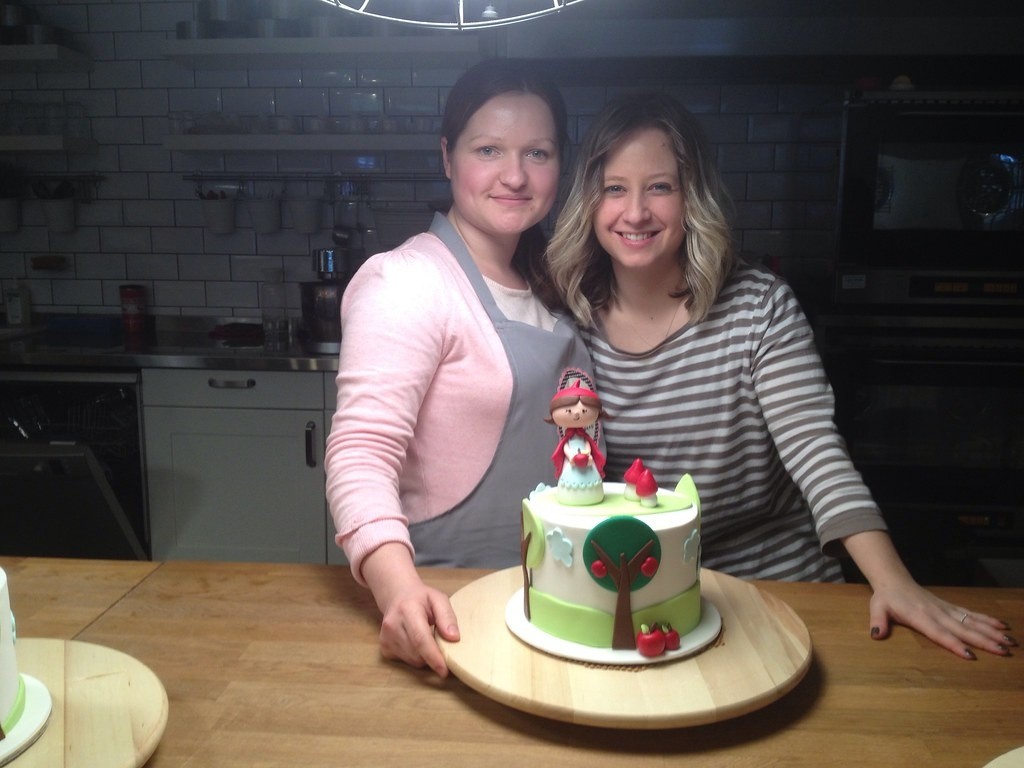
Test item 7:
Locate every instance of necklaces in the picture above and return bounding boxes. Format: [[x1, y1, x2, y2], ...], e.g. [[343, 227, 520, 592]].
[[616, 277, 681, 349]]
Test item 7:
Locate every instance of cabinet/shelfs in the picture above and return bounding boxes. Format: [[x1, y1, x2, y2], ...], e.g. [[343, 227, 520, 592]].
[[0, 46, 98, 155], [140, 368, 350, 567], [162, 36, 480, 150]]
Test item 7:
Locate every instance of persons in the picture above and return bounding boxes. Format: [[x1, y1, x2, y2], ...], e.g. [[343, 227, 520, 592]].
[[540, 92, 1015, 664], [543, 379, 615, 505], [323, 60, 607, 680]]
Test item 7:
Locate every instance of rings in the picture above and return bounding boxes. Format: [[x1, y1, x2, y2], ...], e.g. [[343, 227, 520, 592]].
[[960, 613, 968, 622]]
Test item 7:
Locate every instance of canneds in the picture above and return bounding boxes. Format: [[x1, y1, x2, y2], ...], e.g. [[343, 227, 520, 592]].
[[118, 285, 144, 334]]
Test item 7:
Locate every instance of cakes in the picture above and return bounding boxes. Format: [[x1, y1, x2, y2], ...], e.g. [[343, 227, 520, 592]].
[[0, 565, 25, 742], [520, 379, 702, 657]]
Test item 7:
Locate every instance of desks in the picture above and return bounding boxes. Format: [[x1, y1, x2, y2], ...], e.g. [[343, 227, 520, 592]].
[[1, 555, 1024, 766]]
